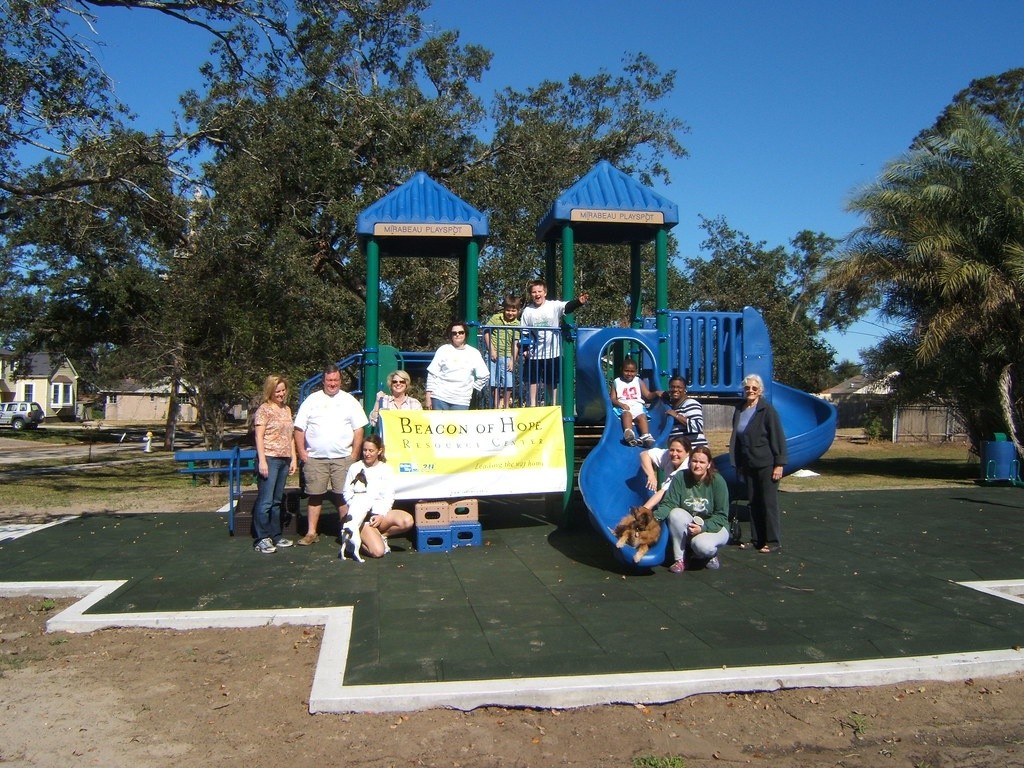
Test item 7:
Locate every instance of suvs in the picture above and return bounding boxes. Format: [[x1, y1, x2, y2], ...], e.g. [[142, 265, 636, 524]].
[[0, 401, 44, 432]]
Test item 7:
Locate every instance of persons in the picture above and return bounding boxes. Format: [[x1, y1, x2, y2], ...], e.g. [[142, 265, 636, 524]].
[[729, 374, 787, 553], [653, 446, 729, 573], [294, 364, 369, 546], [484, 294, 521, 409], [611, 360, 669, 447], [252, 375, 297, 553], [665, 375, 709, 449], [342, 435, 413, 558], [426, 321, 490, 410], [640, 437, 692, 510], [520, 280, 588, 406], [369, 370, 423, 427]]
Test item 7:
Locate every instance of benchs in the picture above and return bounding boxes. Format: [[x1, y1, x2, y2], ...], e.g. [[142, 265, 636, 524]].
[[174, 449, 258, 487]]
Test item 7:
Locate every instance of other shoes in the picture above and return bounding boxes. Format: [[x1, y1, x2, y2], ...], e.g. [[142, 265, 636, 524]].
[[382, 535, 391, 554], [624, 429, 637, 446], [637, 434, 655, 447], [670, 560, 690, 572], [706, 556, 720, 569]]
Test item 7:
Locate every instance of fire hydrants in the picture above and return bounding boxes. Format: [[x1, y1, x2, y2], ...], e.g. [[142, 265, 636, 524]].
[[143, 431, 153, 453]]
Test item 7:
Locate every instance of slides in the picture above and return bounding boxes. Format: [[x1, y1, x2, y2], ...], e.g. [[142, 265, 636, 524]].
[[711, 382, 837, 502], [583, 392, 669, 570]]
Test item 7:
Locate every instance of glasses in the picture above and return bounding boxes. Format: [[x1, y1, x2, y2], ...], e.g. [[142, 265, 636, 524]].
[[744, 386, 758, 391], [392, 380, 406, 384], [450, 331, 463, 336]]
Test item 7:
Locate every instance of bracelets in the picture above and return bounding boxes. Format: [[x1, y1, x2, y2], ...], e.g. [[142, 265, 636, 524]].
[[675, 414, 678, 419], [373, 411, 378, 412]]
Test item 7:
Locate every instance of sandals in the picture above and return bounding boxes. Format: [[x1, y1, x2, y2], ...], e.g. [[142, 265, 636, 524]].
[[739, 542, 757, 548], [760, 545, 782, 552]]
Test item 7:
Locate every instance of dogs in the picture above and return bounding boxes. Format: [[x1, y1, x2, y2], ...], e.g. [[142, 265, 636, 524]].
[[607, 506, 662, 563], [340, 468, 381, 563]]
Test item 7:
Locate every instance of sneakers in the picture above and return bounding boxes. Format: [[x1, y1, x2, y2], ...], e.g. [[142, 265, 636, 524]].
[[298, 534, 319, 545], [276, 539, 293, 547], [255, 538, 277, 554]]
